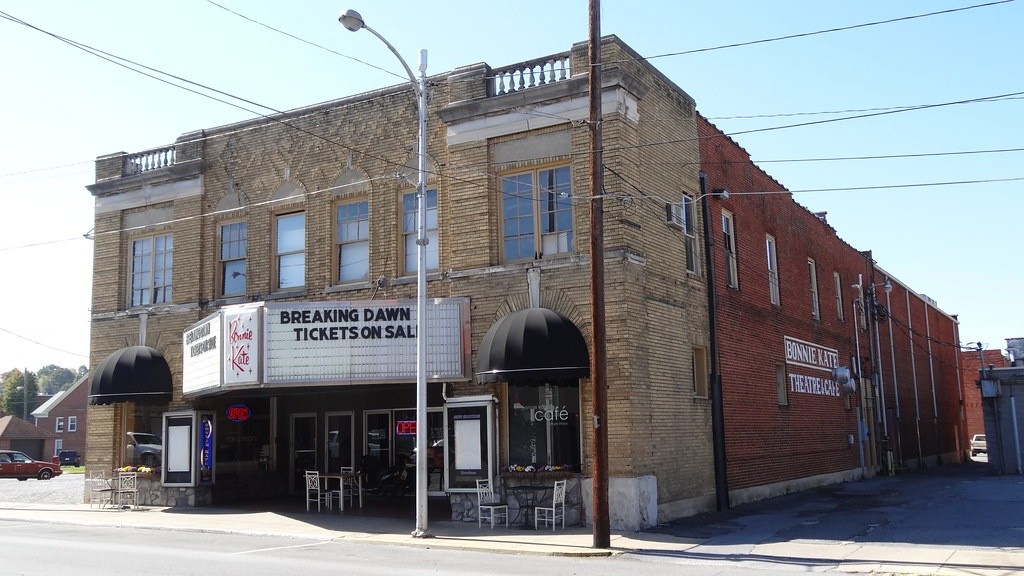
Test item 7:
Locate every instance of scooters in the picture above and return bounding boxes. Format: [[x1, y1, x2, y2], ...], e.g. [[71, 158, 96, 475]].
[[349, 455, 409, 497]]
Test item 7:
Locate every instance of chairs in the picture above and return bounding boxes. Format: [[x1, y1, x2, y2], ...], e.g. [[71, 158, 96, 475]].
[[89, 470, 115, 509], [306, 470, 333, 512], [476, 479, 509, 529], [116, 472, 138, 509], [327, 466, 354, 510], [535, 479, 566, 531]]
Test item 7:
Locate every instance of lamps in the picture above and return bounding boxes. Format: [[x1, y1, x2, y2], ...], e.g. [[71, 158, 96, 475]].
[[713, 189, 730, 201]]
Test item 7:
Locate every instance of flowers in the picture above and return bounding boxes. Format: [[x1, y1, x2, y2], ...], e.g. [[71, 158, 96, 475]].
[[500, 463, 573, 472], [111, 466, 160, 473]]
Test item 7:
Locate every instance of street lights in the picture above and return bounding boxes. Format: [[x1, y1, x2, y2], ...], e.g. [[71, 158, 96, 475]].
[[339, 9, 428, 529]]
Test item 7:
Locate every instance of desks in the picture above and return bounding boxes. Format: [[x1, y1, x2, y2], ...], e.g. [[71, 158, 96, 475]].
[[302, 472, 366, 512], [502, 486, 554, 530]]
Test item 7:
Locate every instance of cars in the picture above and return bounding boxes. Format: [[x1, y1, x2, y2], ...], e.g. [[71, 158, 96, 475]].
[[127, 432, 163, 468], [405, 446, 455, 472], [970, 435, 988, 456], [329, 431, 338, 458], [58, 450, 81, 467], [0, 450, 62, 481], [368, 433, 380, 456]]
